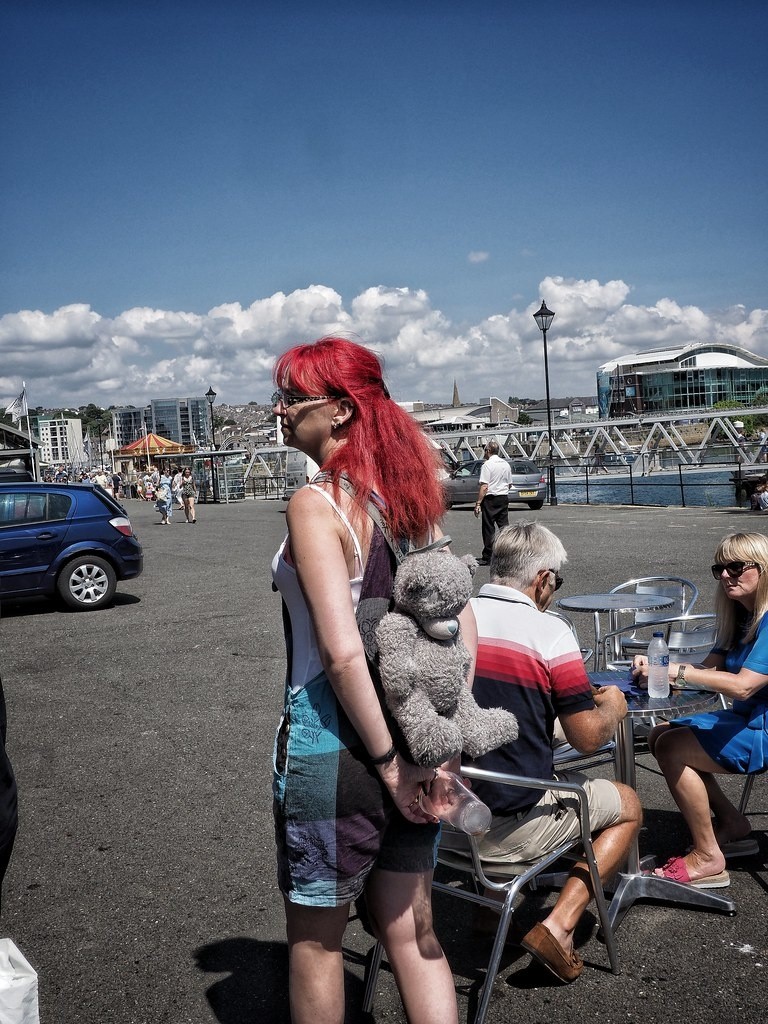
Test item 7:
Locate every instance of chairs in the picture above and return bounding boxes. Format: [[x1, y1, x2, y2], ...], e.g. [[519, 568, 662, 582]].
[[362, 574, 768, 1024], [516, 467, 525, 474]]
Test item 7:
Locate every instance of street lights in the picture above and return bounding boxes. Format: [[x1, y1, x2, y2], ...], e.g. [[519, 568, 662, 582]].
[[533, 299, 559, 505], [205, 386, 220, 503]]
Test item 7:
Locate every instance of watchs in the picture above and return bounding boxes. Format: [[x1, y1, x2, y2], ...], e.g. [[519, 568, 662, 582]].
[[674, 666, 686, 688]]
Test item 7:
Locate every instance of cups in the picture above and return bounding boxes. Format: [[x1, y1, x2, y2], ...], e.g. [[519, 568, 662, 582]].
[[419, 771, 492, 836]]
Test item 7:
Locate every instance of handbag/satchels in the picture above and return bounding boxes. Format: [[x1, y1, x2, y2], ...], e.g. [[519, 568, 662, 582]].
[[156, 489, 168, 502]]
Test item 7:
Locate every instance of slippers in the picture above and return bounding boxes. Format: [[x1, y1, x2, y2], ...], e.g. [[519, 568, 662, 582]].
[[682, 831, 759, 858], [651, 856, 731, 888]]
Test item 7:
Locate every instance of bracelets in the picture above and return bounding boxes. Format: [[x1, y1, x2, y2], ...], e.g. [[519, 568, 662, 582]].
[[476, 503, 481, 506], [373, 745, 398, 765]]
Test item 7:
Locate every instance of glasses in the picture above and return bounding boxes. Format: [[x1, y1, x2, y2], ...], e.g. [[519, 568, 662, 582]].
[[270, 386, 356, 410], [538, 566, 563, 591], [710, 560, 759, 581], [484, 447, 491, 452]]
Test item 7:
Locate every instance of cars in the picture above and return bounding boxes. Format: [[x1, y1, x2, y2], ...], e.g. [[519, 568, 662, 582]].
[[0, 482, 144, 612], [439, 457, 550, 510]]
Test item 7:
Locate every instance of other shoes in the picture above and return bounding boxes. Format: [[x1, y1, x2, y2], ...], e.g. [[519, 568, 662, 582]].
[[475, 559, 490, 565], [161, 518, 166, 525], [520, 921, 584, 984], [166, 519, 171, 525]]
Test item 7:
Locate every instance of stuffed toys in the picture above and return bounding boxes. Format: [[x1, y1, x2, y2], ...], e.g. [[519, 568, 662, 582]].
[[377, 549, 519, 767]]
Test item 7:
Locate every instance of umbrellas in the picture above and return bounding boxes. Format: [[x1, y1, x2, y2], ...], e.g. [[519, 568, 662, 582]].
[[118, 433, 184, 471]]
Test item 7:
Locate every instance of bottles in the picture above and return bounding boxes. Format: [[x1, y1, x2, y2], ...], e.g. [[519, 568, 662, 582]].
[[647, 632, 670, 698]]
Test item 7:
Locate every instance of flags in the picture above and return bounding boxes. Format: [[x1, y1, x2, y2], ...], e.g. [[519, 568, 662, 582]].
[[3, 388, 27, 424]]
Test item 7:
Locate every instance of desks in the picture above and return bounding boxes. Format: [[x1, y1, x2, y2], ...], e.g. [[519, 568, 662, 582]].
[[584, 670, 736, 941], [556, 594, 676, 659]]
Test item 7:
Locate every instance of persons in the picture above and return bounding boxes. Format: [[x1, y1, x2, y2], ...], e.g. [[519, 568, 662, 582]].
[[629, 532, 767, 888], [475, 441, 514, 565], [112, 465, 197, 525], [467, 518, 642, 985], [105, 472, 112, 486], [45, 466, 108, 490], [268, 338, 459, 1024]]
